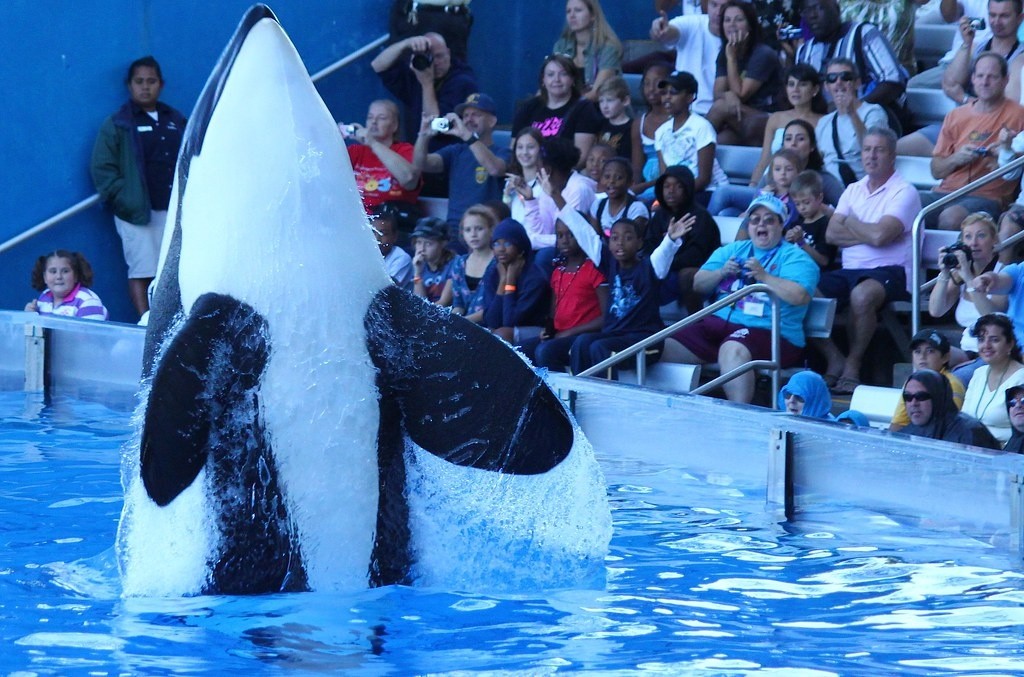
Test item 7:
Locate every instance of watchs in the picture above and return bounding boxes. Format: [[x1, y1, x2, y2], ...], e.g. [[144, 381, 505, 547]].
[[466, 131, 480, 145]]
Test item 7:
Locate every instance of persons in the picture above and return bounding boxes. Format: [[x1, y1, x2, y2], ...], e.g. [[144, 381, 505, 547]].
[[338, 0, 1023, 453], [90, 56, 189, 317], [24, 251, 109, 324]]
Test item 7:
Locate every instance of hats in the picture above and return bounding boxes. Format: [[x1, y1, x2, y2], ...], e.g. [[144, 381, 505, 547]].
[[658, 71, 697, 95], [909, 328, 949, 352], [746, 194, 788, 222], [454, 93, 497, 117], [410, 216, 448, 240], [489, 219, 529, 250]]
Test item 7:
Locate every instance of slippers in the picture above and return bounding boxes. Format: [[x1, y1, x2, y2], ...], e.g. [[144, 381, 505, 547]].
[[831, 377, 861, 394], [823, 375, 839, 387]]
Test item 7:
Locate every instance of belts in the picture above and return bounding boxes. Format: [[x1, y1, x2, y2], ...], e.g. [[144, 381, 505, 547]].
[[408, 4, 467, 14]]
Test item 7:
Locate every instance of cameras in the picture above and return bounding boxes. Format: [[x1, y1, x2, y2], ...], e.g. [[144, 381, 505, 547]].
[[974, 148, 989, 158], [967, 16, 986, 30], [342, 125, 355, 137], [412, 50, 434, 71], [431, 117, 450, 133], [779, 25, 803, 41], [733, 259, 757, 285], [941, 240, 973, 269]]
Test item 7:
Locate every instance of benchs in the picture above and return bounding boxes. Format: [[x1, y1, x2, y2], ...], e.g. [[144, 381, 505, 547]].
[[416, 25, 963, 427]]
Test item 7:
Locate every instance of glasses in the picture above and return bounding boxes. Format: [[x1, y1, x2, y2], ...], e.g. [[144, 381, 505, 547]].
[[904, 391, 944, 401], [783, 390, 804, 403], [544, 52, 572, 60], [1008, 398, 1024, 406], [492, 242, 512, 248], [826, 71, 857, 83], [748, 213, 781, 225]]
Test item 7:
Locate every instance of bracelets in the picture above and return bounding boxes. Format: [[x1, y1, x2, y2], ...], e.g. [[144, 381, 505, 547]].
[[413, 277, 423, 282], [961, 93, 969, 104], [937, 271, 951, 282], [505, 285, 516, 291]]
